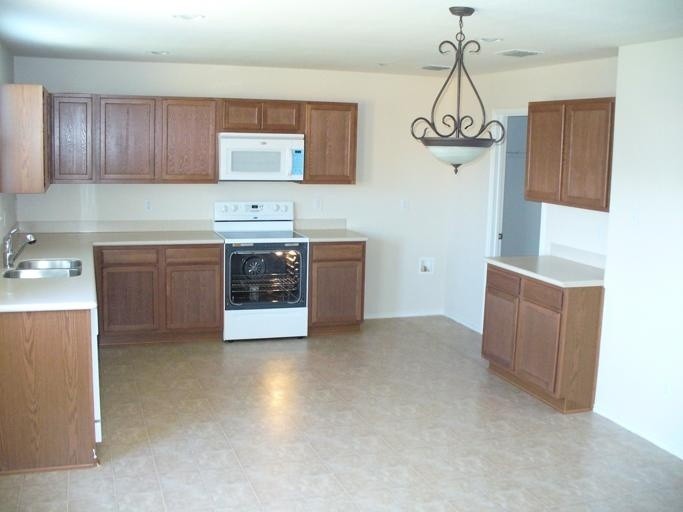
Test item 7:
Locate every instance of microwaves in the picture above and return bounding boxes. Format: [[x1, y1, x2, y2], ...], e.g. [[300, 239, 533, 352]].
[[217, 132, 305, 181]]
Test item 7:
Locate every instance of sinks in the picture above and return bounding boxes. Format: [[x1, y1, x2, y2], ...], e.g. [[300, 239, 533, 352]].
[[16, 259, 82, 269], [3, 270, 81, 279]]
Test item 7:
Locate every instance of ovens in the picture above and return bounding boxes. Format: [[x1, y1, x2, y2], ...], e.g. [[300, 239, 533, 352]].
[[223, 250, 310, 343]]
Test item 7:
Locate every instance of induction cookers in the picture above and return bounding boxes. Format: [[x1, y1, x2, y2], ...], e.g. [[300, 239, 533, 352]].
[[215, 230, 308, 250]]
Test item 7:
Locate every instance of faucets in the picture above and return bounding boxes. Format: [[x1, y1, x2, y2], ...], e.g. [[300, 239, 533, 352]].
[[3, 227, 37, 267]]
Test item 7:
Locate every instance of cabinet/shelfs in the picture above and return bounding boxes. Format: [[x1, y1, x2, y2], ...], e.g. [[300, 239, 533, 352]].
[[293, 100, 357, 185], [521, 96, 614, 213], [214, 98, 306, 133], [93, 245, 224, 348], [483, 263, 604, 415], [0, 84, 50, 195], [48, 93, 96, 184], [308, 242, 366, 337], [92, 95, 220, 184]]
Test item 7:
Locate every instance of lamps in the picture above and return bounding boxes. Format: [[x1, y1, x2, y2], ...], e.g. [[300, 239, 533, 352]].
[[410, 5, 504, 175]]
